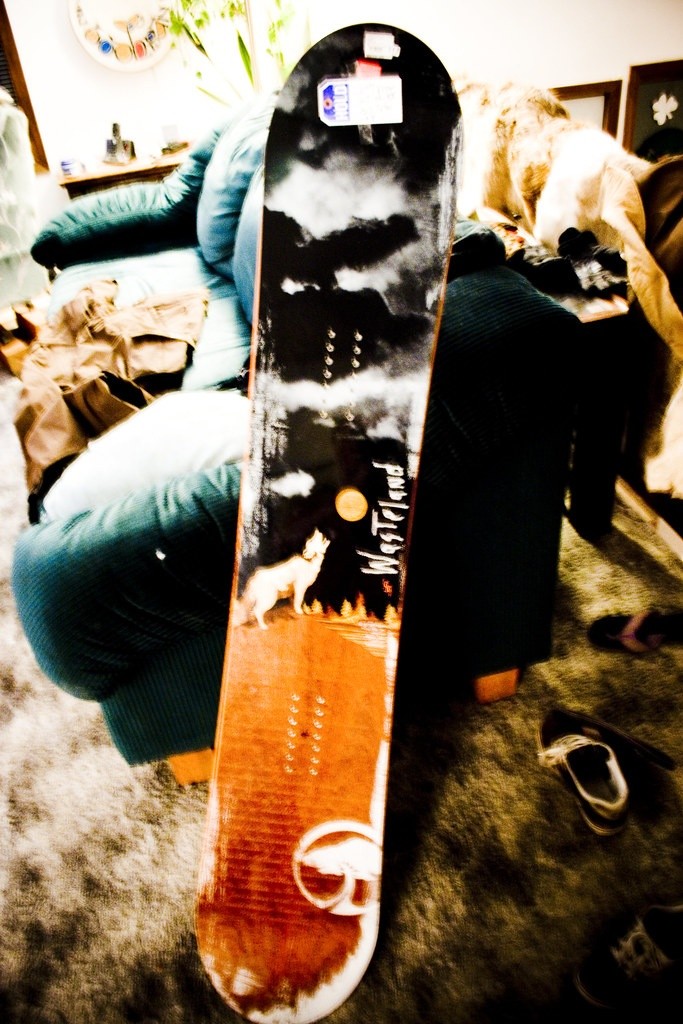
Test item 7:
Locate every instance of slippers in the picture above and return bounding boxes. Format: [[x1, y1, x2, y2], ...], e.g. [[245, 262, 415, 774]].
[[588, 609, 683, 654]]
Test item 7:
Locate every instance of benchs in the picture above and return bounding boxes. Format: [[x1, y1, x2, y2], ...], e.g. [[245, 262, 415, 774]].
[[517, 60, 683, 541]]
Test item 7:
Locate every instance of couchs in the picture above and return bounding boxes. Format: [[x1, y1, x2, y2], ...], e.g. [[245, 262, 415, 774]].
[[13, 107, 582, 782]]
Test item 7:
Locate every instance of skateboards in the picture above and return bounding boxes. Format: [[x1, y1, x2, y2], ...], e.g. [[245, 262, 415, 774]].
[[193, 22, 464, 1024]]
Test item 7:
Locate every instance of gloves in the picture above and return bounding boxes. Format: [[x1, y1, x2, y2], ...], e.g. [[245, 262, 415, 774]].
[[558, 228, 627, 293]]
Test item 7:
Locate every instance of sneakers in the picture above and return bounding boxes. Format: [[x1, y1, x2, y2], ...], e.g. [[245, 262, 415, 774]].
[[537, 710, 629, 835]]
